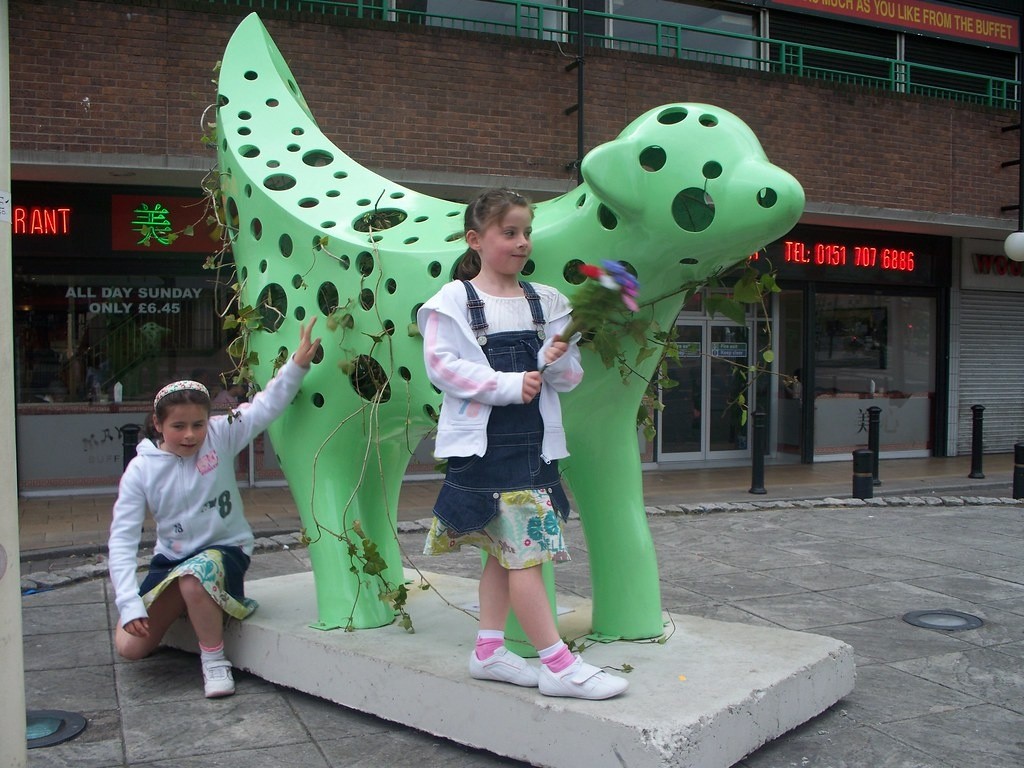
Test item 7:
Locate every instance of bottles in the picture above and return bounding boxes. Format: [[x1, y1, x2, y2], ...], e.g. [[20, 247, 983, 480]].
[[87, 392, 96, 407], [85, 366, 105, 401], [113, 381, 123, 403], [865, 375, 889, 394], [783, 376, 803, 400]]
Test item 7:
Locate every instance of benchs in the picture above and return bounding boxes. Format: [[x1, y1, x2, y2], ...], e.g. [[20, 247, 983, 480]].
[[778, 390, 936, 454]]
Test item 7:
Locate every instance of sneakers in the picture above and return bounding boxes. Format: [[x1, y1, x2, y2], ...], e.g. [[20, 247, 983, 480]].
[[202, 658, 235, 698], [539, 654, 630, 699], [469, 645, 540, 688]]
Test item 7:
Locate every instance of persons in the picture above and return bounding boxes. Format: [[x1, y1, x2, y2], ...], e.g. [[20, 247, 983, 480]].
[[417, 187, 628, 700], [107, 315, 322, 699]]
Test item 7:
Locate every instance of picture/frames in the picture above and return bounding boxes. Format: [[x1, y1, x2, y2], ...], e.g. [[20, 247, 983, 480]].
[[815, 306, 887, 369]]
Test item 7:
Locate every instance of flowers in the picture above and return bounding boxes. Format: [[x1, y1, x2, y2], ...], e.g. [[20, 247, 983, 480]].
[[540, 258, 640, 375]]
